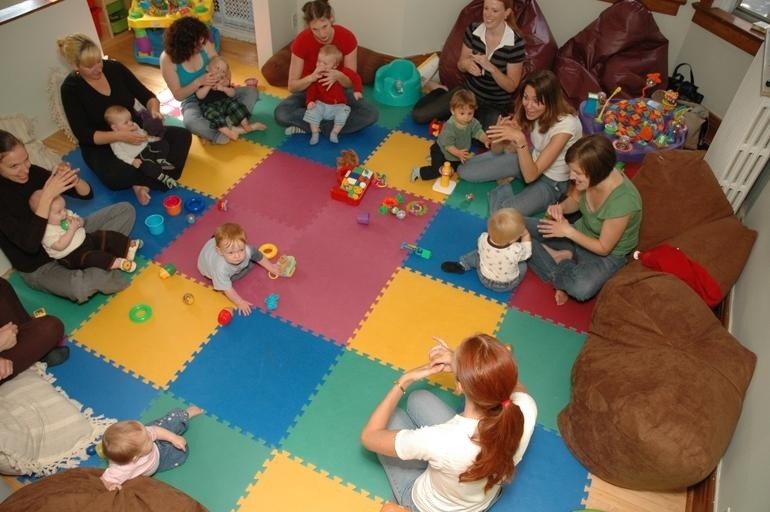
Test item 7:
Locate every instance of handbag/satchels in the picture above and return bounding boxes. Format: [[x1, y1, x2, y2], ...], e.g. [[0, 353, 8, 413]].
[[668, 62, 704, 104], [652, 88, 709, 150]]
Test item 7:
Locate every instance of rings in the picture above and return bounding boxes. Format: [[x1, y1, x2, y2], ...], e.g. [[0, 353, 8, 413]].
[[469, 71, 472, 74]]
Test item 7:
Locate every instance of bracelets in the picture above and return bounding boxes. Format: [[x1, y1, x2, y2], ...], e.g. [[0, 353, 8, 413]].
[[394, 381, 405, 395], [515, 143, 528, 150]]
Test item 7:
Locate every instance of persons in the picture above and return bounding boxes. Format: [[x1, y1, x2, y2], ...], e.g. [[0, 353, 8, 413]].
[[359, 334, 538, 512], [197, 222, 283, 317], [413, 1, 528, 148], [0, 278, 70, 385], [0, 129, 144, 305], [303, 45, 364, 147], [56, 34, 193, 207], [514, 134, 644, 306], [160, 15, 268, 147], [196, 57, 253, 141], [98, 404, 204, 491], [408, 90, 491, 182], [104, 105, 180, 190], [274, 1, 379, 140], [28, 191, 141, 273], [441, 207, 532, 294], [456, 68, 584, 219]]
